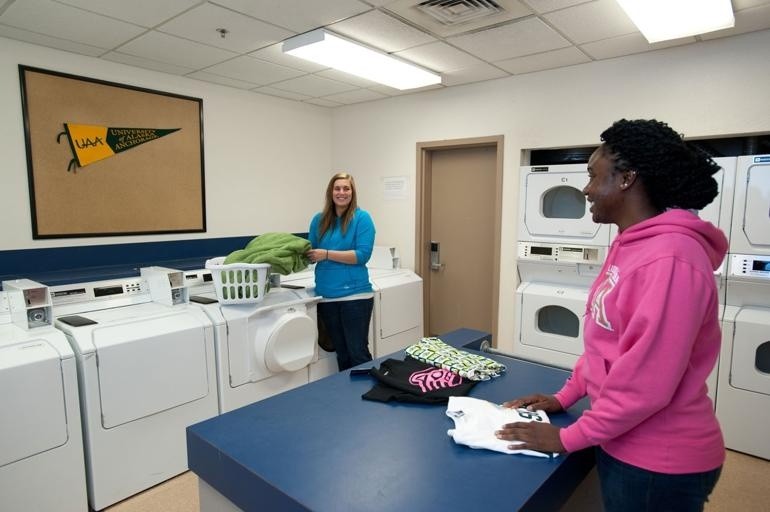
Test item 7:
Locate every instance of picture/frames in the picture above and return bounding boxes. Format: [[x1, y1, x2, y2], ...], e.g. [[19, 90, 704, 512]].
[[18, 64, 206, 240]]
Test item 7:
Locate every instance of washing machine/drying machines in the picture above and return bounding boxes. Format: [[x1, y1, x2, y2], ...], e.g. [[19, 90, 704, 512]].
[[512, 154, 770, 461], [0, 247, 424, 511]]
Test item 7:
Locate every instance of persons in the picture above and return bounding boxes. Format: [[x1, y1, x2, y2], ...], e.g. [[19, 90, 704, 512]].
[[308, 172, 376, 373], [495, 116, 729, 512]]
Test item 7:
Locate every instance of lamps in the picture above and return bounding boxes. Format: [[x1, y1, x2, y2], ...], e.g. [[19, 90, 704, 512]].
[[615, 0, 736, 44], [282, 28, 441, 91]]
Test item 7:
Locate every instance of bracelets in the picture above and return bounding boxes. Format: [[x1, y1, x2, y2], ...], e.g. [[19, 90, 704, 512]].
[[326, 249, 328, 260]]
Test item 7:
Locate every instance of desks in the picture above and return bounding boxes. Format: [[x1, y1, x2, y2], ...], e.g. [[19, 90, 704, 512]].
[[186, 329, 600, 512]]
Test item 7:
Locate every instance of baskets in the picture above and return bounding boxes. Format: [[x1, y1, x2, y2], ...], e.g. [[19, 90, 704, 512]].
[[206, 254, 271, 305]]
